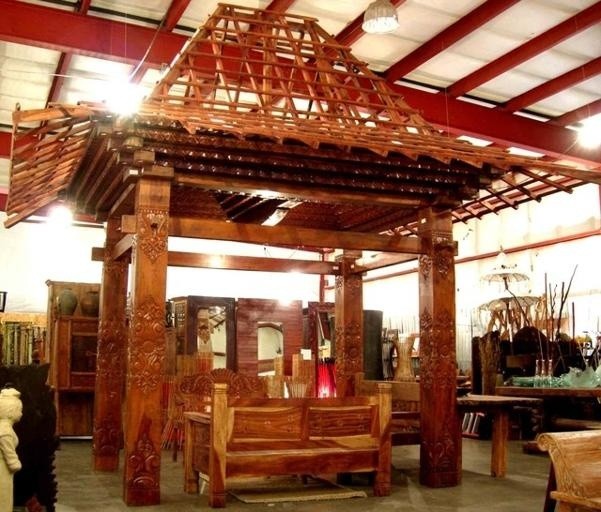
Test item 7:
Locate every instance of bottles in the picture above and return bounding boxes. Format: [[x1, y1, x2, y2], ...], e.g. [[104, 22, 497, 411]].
[[57, 286, 77, 316], [80, 290, 100, 318]]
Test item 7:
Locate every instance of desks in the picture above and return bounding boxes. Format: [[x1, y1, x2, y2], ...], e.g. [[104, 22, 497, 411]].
[[456, 395, 543, 479]]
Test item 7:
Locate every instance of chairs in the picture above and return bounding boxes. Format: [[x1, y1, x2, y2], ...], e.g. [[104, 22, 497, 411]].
[[536, 429, 600, 512]]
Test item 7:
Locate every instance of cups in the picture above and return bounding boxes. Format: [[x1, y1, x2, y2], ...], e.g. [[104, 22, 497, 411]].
[[534, 358, 554, 389]]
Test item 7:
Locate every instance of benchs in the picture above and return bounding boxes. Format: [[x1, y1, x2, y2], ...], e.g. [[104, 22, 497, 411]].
[[182, 381, 397, 510]]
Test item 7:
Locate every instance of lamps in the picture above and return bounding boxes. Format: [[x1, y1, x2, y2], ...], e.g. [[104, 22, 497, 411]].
[[361, 1, 400, 34]]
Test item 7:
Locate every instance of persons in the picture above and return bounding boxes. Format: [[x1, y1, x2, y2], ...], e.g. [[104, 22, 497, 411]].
[[1, 385, 26, 511]]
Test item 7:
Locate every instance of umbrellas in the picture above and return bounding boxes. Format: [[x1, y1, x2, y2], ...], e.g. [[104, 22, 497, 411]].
[[484, 245, 530, 291], [480, 291, 544, 356]]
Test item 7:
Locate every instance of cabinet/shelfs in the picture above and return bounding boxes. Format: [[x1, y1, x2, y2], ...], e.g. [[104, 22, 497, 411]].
[[44, 281, 102, 439]]
